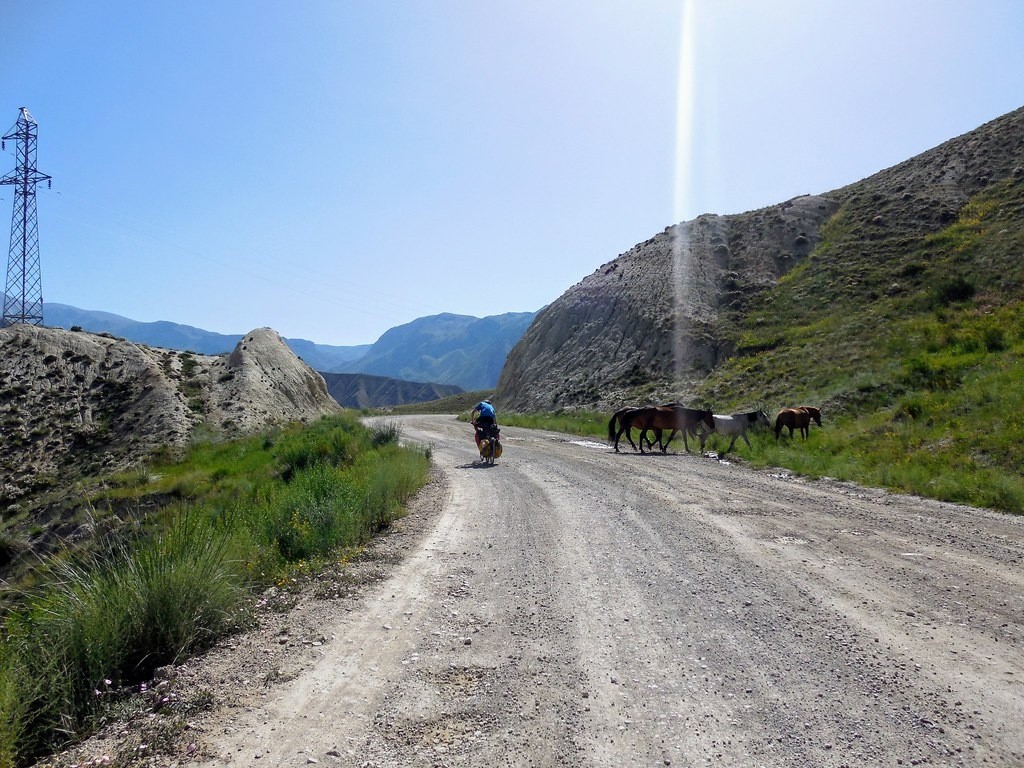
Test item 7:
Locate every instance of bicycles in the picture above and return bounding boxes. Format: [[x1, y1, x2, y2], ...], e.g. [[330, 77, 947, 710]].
[[471, 422, 502, 465]]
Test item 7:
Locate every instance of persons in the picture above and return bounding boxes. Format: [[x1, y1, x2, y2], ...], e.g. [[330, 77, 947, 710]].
[[471, 400, 498, 446]]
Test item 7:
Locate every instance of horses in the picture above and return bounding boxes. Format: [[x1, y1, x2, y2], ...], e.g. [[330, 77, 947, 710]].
[[608, 399, 823, 455]]
